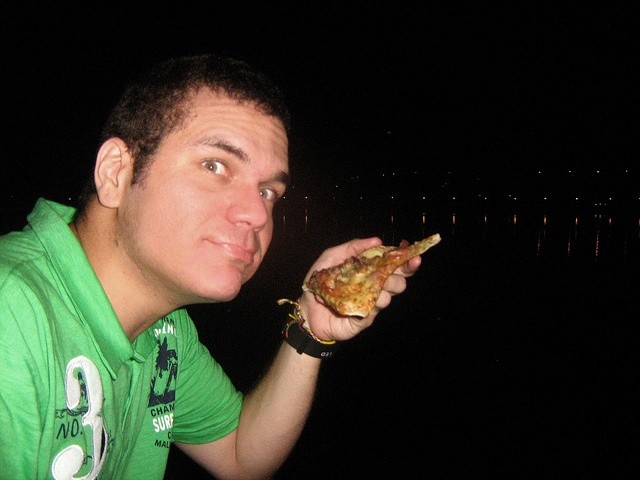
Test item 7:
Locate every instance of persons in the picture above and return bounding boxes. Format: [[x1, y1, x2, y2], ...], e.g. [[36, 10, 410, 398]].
[[1, 52, 422, 479]]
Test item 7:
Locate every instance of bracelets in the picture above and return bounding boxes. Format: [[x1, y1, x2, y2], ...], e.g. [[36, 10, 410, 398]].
[[280, 318, 343, 361]]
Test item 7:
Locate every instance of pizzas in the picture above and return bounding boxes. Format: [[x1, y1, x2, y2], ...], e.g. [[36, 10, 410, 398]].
[[305, 234, 441, 319]]
